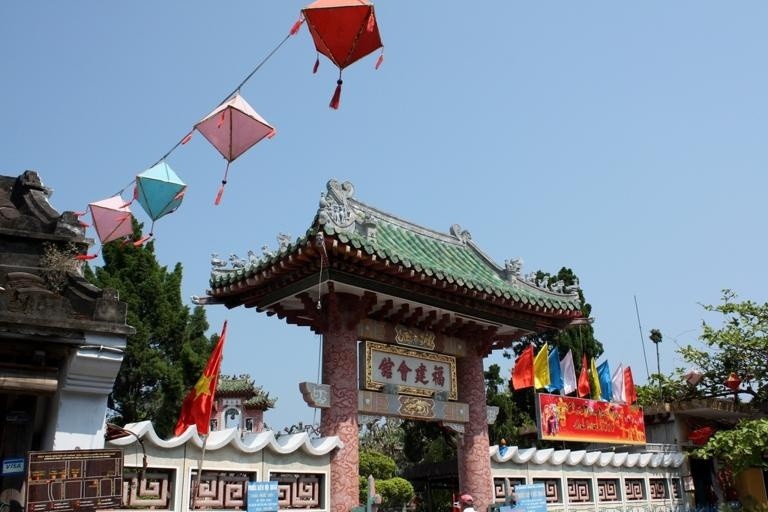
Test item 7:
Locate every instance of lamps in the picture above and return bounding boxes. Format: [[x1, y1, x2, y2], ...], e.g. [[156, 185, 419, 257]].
[[316, 255, 323, 310]]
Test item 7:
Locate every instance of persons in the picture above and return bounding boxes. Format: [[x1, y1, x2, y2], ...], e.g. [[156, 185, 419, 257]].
[[460, 493, 477, 512]]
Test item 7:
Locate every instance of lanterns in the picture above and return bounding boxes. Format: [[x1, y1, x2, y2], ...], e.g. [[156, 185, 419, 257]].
[[290, 1, 387, 111], [120, 161, 190, 248], [685, 368, 704, 387], [70, 193, 135, 260], [724, 372, 741, 389], [181, 92, 277, 209]]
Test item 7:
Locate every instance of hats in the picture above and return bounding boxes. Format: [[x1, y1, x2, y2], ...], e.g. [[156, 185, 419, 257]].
[[458, 495, 474, 505]]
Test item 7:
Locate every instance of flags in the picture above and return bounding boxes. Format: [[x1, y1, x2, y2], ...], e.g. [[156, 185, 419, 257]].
[[559, 347, 578, 396], [595, 360, 613, 403], [534, 340, 550, 389], [512, 343, 534, 389], [577, 352, 590, 397], [611, 360, 626, 403], [588, 356, 603, 400], [173, 319, 228, 438], [546, 346, 563, 392], [623, 366, 637, 405]]
